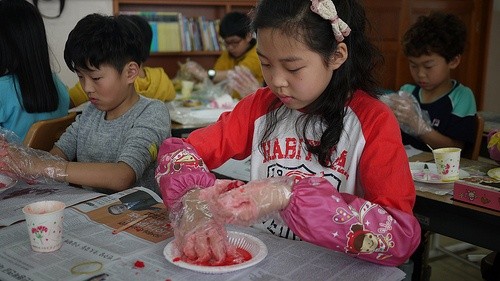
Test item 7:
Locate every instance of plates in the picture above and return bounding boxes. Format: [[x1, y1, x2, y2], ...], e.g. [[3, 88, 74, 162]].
[[488, 168, 500, 180], [162, 230, 269, 274], [0, 172, 18, 194], [409, 162, 469, 184]]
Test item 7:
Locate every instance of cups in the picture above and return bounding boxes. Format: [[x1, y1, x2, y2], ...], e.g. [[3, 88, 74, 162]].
[[22, 200, 66, 253], [432, 147, 462, 181], [181, 80, 194, 98]]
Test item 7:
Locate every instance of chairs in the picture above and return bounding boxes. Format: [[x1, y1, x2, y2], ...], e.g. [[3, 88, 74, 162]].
[[471, 112, 485, 161], [22, 113, 78, 147]]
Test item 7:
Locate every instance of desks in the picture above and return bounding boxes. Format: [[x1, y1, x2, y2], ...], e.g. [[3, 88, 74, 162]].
[[0, 121, 500, 281]]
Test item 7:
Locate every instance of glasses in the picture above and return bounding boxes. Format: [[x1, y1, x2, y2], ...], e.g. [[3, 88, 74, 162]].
[[222, 38, 243, 48]]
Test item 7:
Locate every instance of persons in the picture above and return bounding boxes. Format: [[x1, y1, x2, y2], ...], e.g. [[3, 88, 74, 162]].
[[0, 0, 176, 267], [108, 191, 159, 216], [176, 12, 265, 100], [153, 0, 419, 266], [226, 15, 478, 158]]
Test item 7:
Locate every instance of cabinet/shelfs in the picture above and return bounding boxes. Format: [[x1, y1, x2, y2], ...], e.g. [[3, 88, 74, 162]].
[[113, 0, 494, 110]]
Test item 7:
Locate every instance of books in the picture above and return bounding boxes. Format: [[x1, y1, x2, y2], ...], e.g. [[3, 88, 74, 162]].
[[180, 16, 225, 53]]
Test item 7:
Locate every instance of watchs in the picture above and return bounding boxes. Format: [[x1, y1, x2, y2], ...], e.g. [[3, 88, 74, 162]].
[[207, 69, 215, 80]]
